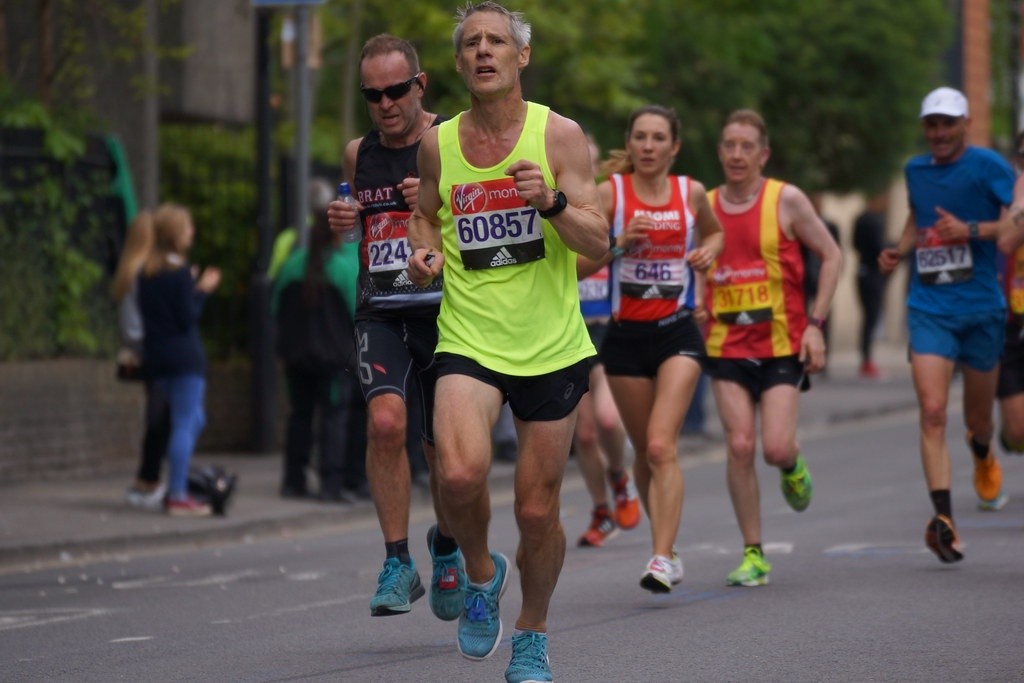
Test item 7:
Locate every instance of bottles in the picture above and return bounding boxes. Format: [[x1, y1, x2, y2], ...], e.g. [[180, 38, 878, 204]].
[[337, 181, 363, 244]]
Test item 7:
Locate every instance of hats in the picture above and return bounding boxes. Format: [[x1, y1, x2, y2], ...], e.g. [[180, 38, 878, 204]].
[[918, 86, 970, 122]]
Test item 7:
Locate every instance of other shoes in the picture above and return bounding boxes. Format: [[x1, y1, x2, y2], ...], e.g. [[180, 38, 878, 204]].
[[860, 364, 880, 378], [164, 495, 214, 518], [125, 482, 166, 508], [281, 478, 374, 508]]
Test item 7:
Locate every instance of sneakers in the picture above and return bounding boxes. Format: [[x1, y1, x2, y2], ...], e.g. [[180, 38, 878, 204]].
[[456, 551, 510, 661], [578, 506, 619, 548], [605, 465, 640, 529], [924, 514, 964, 564], [726, 544, 770, 587], [668, 545, 682, 586], [370, 551, 424, 616], [426, 522, 468, 621], [639, 556, 673, 594], [779, 454, 812, 511], [504, 629, 554, 683], [965, 430, 1003, 503]]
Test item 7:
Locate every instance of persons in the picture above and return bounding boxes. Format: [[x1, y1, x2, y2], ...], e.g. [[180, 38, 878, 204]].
[[696, 109, 843, 587], [303, 35, 466, 623], [405, 0, 607, 683], [107, 180, 1024, 521], [877, 87, 1024, 563], [573, 106, 726, 593]]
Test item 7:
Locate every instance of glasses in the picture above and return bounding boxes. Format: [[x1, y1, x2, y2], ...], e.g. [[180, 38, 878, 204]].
[[358, 73, 421, 103]]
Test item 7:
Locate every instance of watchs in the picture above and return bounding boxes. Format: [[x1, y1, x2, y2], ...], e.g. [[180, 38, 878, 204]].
[[537, 189, 568, 219]]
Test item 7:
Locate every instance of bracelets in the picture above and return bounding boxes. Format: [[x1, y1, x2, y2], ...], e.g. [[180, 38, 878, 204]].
[[610, 247, 625, 257], [966, 220, 978, 242], [807, 316, 826, 329]]
